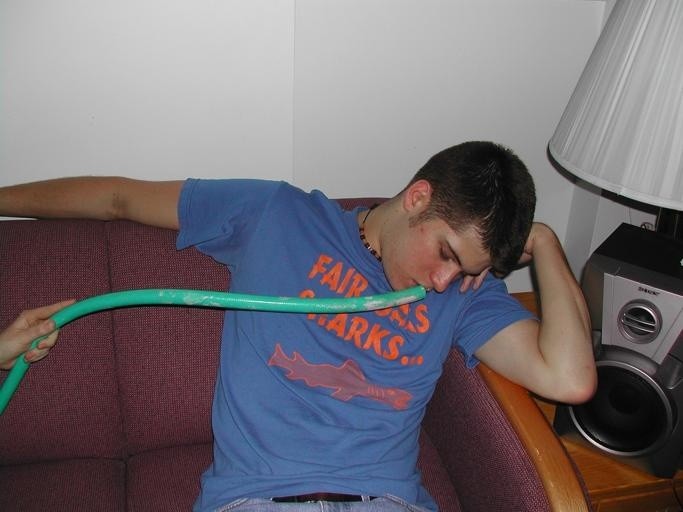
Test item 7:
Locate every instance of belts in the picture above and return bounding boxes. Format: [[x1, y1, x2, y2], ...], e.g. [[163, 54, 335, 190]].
[[272, 492, 378, 503]]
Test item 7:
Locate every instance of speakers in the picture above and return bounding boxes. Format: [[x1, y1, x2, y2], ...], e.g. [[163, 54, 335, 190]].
[[551, 221, 683, 480]]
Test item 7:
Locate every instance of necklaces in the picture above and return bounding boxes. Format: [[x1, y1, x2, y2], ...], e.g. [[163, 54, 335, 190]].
[[358, 203, 382, 263]]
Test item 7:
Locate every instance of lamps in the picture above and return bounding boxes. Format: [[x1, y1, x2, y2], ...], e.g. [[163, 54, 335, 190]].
[[547, 1, 683, 242]]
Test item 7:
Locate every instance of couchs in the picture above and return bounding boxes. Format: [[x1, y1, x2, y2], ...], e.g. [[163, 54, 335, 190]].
[[0, 198, 593, 512]]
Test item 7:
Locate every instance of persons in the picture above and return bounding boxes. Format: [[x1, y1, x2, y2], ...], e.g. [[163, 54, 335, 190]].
[[0, 299, 75, 371], [0, 140, 597, 512]]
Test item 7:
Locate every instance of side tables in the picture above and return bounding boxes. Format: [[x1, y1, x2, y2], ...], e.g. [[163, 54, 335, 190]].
[[508, 290, 683, 512]]
